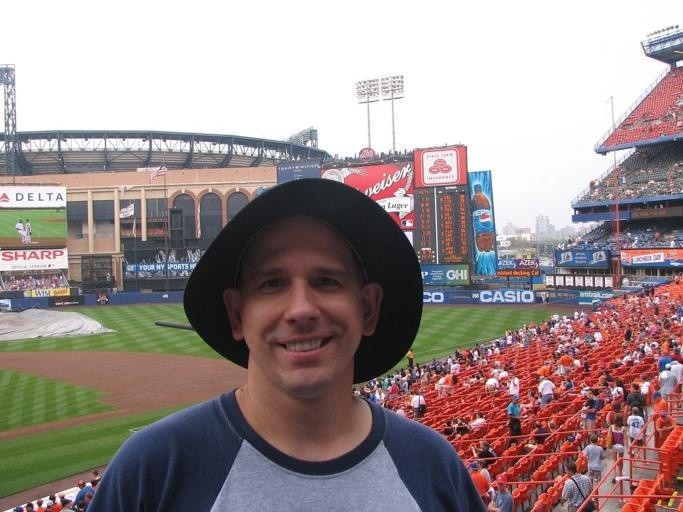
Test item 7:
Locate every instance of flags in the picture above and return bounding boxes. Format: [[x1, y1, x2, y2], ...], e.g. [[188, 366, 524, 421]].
[[150, 165, 169, 183]]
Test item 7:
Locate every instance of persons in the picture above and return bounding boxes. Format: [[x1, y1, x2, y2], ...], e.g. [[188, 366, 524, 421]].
[[665, 94, 683, 131], [575, 150, 683, 203], [2, 470, 101, 512], [352, 276, 682, 511], [1, 275, 68, 291], [82, 176, 485, 511], [557, 223, 683, 250], [16, 217, 34, 245]]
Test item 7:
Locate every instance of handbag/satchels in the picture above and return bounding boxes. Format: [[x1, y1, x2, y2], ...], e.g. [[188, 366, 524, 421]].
[[581, 496, 596, 512]]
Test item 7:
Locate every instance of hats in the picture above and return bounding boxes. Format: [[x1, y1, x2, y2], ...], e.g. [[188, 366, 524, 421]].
[[183, 178, 424, 385], [470, 460, 480, 470], [511, 394, 520, 402]]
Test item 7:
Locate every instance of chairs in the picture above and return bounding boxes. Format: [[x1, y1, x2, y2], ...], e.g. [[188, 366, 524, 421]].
[[379, 277, 683, 509], [567, 63, 682, 251], [0, 271, 70, 291]]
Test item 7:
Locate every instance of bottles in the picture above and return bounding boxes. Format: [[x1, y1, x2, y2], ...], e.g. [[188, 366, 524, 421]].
[[469, 183, 497, 253]]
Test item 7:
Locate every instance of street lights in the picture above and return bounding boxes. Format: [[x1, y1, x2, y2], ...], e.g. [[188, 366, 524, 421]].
[[378, 74, 405, 151], [350, 77, 379, 151]]
